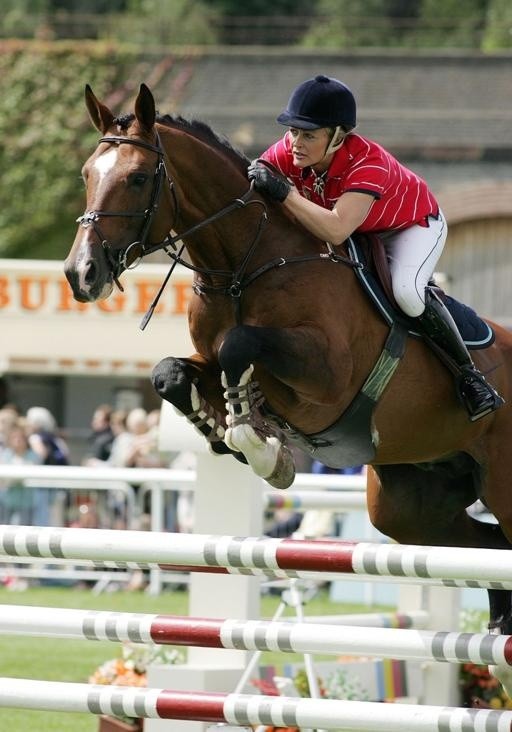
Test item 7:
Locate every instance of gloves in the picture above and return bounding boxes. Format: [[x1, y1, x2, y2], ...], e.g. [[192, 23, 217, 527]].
[[247, 166, 290, 203]]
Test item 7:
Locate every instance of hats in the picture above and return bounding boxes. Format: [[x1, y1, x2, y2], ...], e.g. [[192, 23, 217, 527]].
[[277, 75, 356, 130]]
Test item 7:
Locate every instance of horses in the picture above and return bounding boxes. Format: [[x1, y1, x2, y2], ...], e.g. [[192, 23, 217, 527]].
[[64, 82, 510, 635]]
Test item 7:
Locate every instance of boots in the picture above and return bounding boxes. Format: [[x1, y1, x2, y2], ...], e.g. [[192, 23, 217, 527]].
[[403, 287, 506, 422]]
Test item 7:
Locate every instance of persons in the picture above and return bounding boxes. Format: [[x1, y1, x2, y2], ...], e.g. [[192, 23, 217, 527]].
[[261, 457, 364, 595], [241, 73, 503, 423], [0, 405, 199, 591]]
